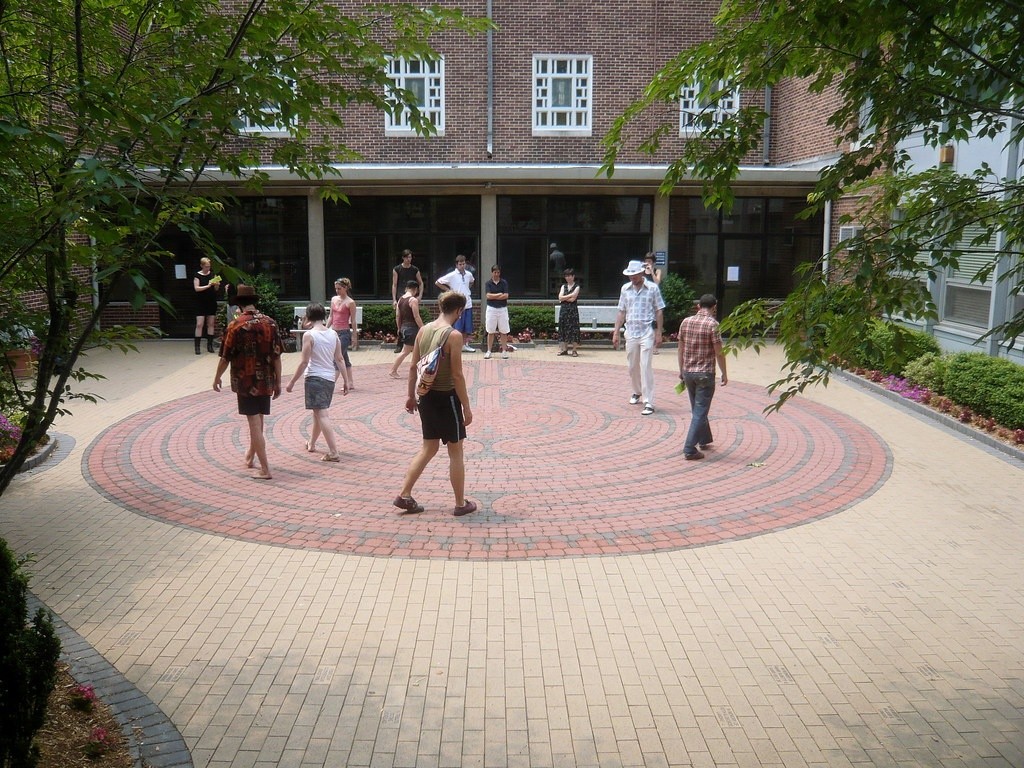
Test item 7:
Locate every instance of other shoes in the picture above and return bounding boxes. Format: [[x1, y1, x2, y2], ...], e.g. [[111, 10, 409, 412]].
[[483, 351, 492, 359], [394, 347, 402, 353], [653, 351, 658, 355], [629, 394, 640, 404], [699, 443, 706, 450], [462, 344, 476, 352], [641, 406, 654, 414], [557, 350, 569, 355], [572, 351, 578, 357], [685, 451, 704, 460], [502, 350, 508, 359], [341, 387, 354, 391]]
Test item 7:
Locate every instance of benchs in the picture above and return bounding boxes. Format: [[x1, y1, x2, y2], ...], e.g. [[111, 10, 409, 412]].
[[291, 306, 364, 350], [555, 305, 628, 347]]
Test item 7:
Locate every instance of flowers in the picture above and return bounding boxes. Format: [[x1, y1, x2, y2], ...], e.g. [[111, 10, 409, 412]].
[[7, 327, 43, 350]]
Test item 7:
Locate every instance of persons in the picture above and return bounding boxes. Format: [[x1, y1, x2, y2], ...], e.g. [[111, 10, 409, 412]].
[[194, 257, 220, 355], [484, 264, 510, 359], [545, 243, 566, 286], [389, 280, 425, 379], [392, 249, 424, 353], [435, 255, 476, 352], [557, 268, 581, 357], [677, 294, 728, 460], [325, 278, 357, 391], [286, 302, 350, 462], [446, 251, 477, 296], [613, 260, 666, 415], [213, 285, 284, 478], [642, 252, 662, 355], [394, 290, 477, 516]]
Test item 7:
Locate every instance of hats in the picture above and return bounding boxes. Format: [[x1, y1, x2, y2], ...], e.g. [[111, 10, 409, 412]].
[[229, 285, 260, 306], [622, 260, 645, 276], [550, 243, 556, 249]]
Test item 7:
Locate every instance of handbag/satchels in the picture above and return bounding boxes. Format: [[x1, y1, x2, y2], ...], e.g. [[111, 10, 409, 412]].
[[416, 328, 455, 396]]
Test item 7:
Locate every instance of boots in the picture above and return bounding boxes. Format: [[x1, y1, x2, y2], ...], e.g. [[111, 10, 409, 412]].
[[194, 337, 202, 354], [207, 335, 215, 353]]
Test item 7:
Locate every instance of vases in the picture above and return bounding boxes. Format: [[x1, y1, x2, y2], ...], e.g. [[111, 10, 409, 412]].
[[3, 347, 34, 381]]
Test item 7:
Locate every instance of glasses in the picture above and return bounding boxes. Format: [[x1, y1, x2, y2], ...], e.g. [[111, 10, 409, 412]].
[[337, 279, 347, 286]]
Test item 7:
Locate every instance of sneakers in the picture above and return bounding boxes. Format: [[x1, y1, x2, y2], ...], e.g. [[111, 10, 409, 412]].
[[393, 494, 424, 513], [454, 499, 478, 518]]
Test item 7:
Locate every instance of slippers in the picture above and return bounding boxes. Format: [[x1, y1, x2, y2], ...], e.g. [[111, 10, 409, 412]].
[[320, 453, 340, 462], [305, 440, 317, 452]]
